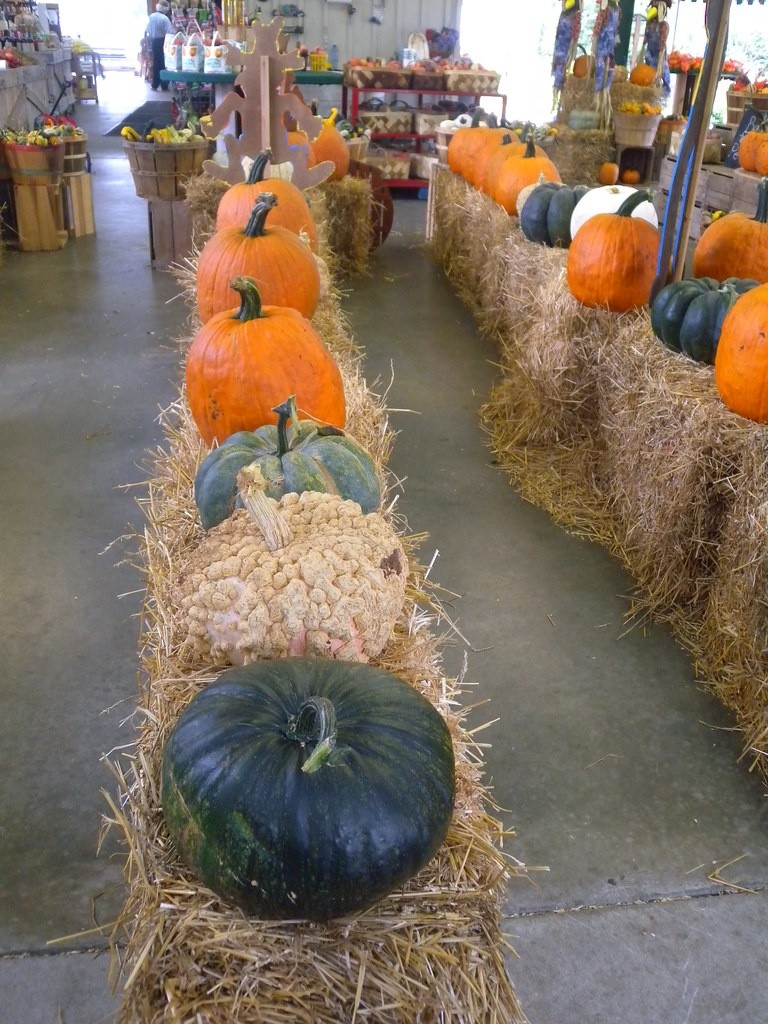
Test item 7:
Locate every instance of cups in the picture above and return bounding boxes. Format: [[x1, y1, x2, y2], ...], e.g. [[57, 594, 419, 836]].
[[402, 48, 419, 67]]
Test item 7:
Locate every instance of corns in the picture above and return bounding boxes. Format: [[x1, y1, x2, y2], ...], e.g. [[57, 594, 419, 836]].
[[573, 8, 669, 51]]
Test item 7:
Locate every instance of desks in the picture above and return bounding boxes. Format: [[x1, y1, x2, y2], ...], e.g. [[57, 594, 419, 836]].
[[160, 69, 343, 139]]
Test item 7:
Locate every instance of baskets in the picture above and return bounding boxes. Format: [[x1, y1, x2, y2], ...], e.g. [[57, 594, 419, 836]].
[[669, 131, 722, 163], [357, 98, 489, 135], [342, 63, 502, 95], [366, 142, 441, 180]]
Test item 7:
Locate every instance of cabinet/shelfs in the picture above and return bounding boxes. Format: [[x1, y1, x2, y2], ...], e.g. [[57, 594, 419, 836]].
[[0, 0, 42, 51], [346, 84, 507, 186]]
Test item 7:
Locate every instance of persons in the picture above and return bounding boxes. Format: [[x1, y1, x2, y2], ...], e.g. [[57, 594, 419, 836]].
[[144, 0, 173, 91]]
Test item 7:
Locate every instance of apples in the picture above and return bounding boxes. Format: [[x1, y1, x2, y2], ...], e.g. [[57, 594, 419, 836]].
[[174, 38, 223, 46], [350, 56, 464, 71]]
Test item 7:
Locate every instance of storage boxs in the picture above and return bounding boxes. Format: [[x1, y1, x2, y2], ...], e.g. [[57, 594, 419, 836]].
[[445, 72, 499, 93], [402, 71, 444, 90], [343, 67, 412, 89], [651, 123, 768, 243]]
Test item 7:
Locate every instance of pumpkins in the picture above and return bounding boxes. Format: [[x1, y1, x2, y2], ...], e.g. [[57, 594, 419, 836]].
[[441, 51, 768, 424], [284, 119, 349, 180], [161, 149, 456, 925]]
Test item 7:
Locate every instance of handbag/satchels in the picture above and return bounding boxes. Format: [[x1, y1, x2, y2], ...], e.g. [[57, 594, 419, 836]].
[[163, 19, 245, 74]]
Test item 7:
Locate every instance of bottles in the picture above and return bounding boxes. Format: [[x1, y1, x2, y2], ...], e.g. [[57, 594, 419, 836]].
[[221, 0, 244, 26], [0, 29, 54, 52], [329, 43, 339, 71], [256, 6, 262, 24]]
